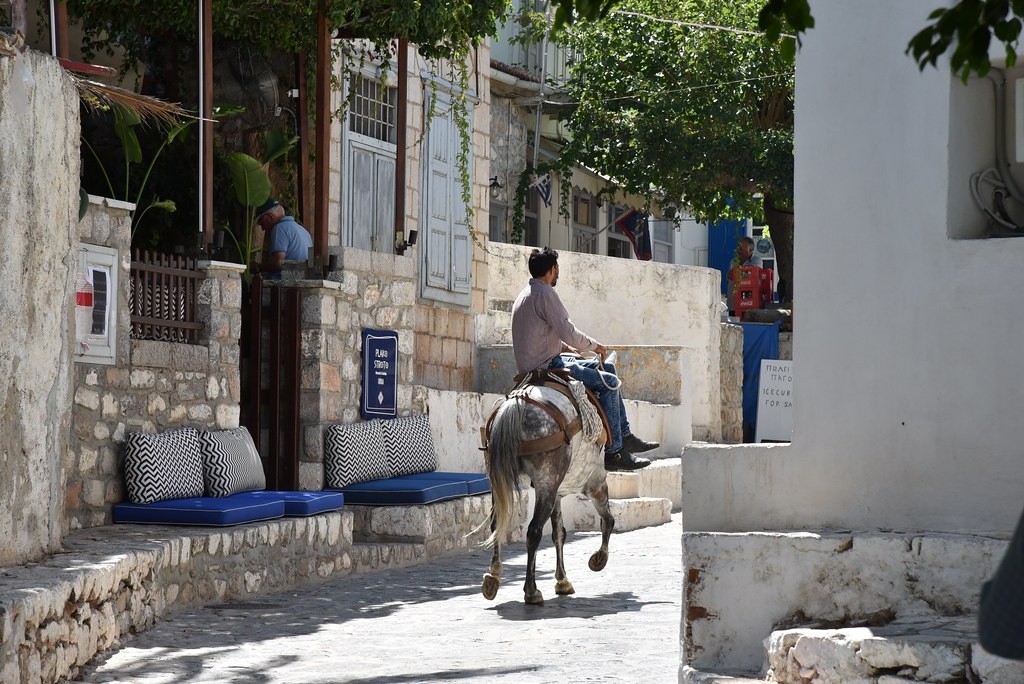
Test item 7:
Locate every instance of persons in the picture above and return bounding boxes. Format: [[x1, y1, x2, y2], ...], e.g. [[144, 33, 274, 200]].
[[511, 247, 661, 471], [727, 235, 764, 315], [248, 196, 316, 279]]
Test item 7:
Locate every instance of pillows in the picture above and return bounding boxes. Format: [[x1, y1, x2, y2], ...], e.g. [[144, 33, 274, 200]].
[[380, 412, 436, 478], [198, 426, 266, 498], [325, 418, 389, 489], [124, 427, 205, 504], [130, 276, 194, 344]]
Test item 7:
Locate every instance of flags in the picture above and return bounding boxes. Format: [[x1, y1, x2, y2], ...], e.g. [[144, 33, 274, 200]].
[[535, 174, 552, 208], [613, 208, 652, 261]]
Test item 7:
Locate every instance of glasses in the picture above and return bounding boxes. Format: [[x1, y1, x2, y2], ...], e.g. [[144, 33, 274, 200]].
[[257, 213, 266, 225]]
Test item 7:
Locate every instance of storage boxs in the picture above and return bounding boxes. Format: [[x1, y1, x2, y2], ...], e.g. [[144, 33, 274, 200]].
[[733, 266, 774, 322]]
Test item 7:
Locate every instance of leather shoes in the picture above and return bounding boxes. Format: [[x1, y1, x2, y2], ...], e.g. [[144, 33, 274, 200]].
[[604, 433, 660, 472]]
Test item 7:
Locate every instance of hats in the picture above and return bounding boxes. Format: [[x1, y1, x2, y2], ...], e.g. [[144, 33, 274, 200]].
[[257, 199, 281, 216]]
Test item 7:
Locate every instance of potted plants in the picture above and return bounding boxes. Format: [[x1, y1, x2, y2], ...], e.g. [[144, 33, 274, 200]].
[[214, 126, 299, 360]]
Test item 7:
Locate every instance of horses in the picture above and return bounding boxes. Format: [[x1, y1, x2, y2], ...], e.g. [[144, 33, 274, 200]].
[[462, 350, 617, 605]]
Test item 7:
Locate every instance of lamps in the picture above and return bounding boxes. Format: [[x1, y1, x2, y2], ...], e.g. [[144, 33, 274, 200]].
[[403, 230, 418, 249], [489, 176, 502, 199]]
